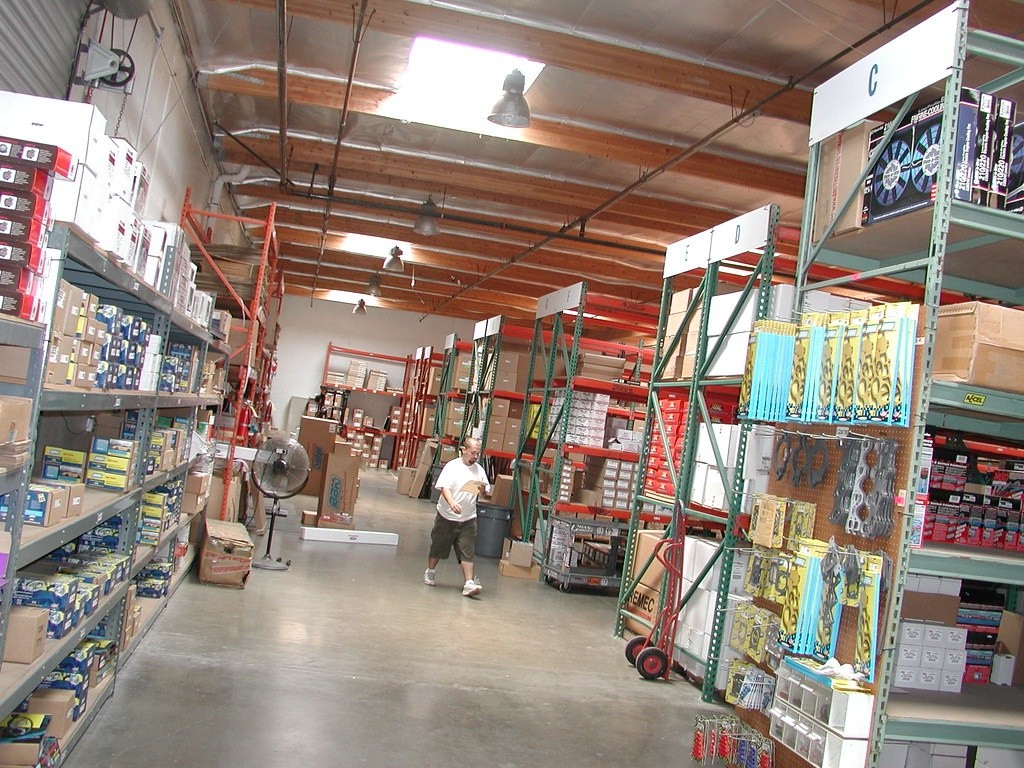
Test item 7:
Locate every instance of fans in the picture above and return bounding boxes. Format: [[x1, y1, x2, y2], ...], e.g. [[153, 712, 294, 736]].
[[252, 437, 311, 569]]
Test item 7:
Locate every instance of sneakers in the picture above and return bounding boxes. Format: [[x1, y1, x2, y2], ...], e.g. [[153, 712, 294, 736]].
[[462, 574, 482, 595], [424, 568, 436, 585]]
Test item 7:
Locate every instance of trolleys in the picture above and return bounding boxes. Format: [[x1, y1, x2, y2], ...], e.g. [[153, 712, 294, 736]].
[[541, 514, 630, 593], [625, 498, 688, 682]]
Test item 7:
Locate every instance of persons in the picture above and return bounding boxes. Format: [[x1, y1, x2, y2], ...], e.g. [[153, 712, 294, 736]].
[[424, 437, 491, 596]]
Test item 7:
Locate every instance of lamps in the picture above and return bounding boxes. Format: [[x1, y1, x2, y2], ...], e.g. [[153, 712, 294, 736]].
[[487, 69, 531, 128], [363, 269, 383, 296], [382, 246, 404, 272], [352, 299, 367, 315], [413, 193, 441, 237]]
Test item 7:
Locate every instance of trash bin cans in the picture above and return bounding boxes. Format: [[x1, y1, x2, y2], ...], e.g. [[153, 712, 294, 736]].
[[474, 502, 515, 559]]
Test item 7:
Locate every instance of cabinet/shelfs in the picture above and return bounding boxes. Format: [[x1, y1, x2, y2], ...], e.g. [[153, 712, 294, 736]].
[[422, 333, 473, 499], [318, 341, 416, 471], [727, 1, 1024, 768], [508, 281, 654, 594], [0, 221, 233, 768], [613, 201, 780, 703], [401, 346, 444, 469], [0, 312, 47, 668], [458, 314, 654, 473], [177, 186, 285, 521]]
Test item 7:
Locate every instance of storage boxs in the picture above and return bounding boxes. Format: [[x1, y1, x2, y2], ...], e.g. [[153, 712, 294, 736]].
[[296, 85, 1023, 767], [0, 88, 280, 768]]
